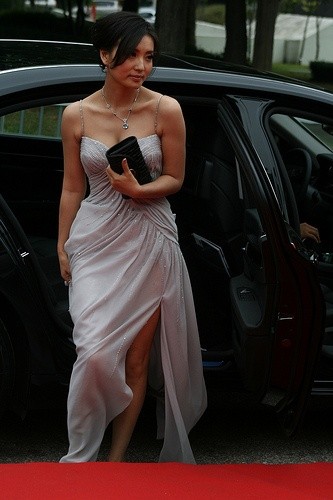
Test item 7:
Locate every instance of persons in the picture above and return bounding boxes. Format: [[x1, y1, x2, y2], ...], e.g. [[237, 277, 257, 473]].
[[57, 12, 207, 465], [300, 222, 320, 244]]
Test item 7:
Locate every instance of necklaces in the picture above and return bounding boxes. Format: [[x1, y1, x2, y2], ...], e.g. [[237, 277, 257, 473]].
[[101, 86, 140, 130]]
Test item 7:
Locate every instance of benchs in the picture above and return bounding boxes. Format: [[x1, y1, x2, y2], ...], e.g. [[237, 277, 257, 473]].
[[0, 191, 74, 324]]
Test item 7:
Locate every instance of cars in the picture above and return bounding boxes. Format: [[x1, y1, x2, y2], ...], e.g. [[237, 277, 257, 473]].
[[1, 0, 333, 437]]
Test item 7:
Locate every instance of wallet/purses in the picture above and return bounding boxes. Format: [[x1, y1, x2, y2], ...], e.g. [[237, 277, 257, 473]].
[[106, 135, 153, 200]]
